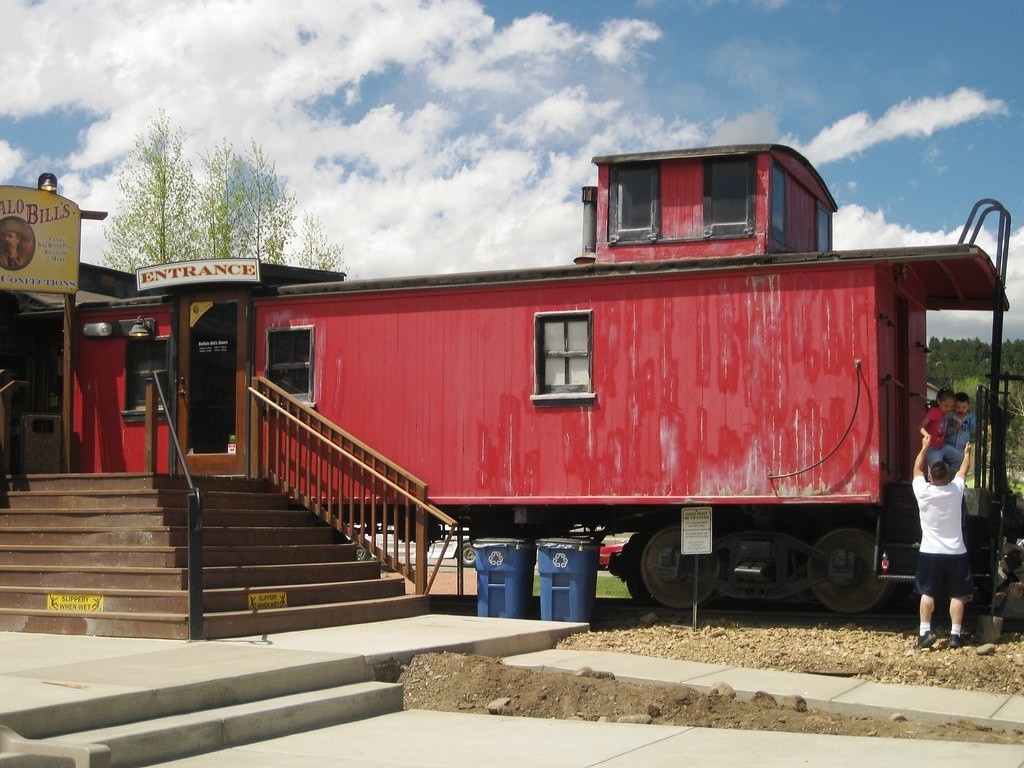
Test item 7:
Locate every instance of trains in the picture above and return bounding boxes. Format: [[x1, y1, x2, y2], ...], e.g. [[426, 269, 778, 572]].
[[0, 144, 1020, 614]]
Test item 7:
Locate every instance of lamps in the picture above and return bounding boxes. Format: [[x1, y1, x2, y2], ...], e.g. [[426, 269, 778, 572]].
[[128, 315, 152, 337]]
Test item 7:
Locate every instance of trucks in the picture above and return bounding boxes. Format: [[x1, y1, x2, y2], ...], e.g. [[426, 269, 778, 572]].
[[344, 522, 479, 567]]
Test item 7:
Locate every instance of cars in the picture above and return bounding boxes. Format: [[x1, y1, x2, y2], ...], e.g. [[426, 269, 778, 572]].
[[600, 537, 629, 567]]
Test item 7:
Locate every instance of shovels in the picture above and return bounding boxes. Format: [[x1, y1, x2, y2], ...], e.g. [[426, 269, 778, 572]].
[[977, 495, 1006, 644]]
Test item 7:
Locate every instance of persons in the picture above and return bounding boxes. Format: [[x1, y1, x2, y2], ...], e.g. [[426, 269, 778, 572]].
[[912, 435, 975, 650], [944, 392, 976, 454], [918, 387, 964, 483], [0, 219, 31, 269]]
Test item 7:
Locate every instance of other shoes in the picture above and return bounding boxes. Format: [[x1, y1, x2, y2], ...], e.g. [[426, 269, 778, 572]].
[[950, 635, 967, 648], [913, 631, 936, 650]]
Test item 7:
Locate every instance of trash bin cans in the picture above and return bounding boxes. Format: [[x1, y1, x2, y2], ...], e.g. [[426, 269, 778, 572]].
[[18, 415, 62, 474], [472, 537, 537, 619], [535, 537, 605, 622]]
